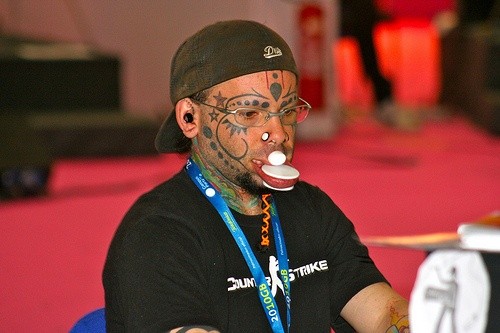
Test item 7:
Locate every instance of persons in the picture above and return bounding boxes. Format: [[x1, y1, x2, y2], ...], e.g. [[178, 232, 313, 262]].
[[101, 19, 411, 333]]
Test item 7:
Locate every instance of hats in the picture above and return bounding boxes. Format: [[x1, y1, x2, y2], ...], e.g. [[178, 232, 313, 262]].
[[153, 19, 298, 155]]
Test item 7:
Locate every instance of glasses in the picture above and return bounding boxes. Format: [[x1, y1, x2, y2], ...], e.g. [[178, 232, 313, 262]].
[[193, 97, 311, 127]]
